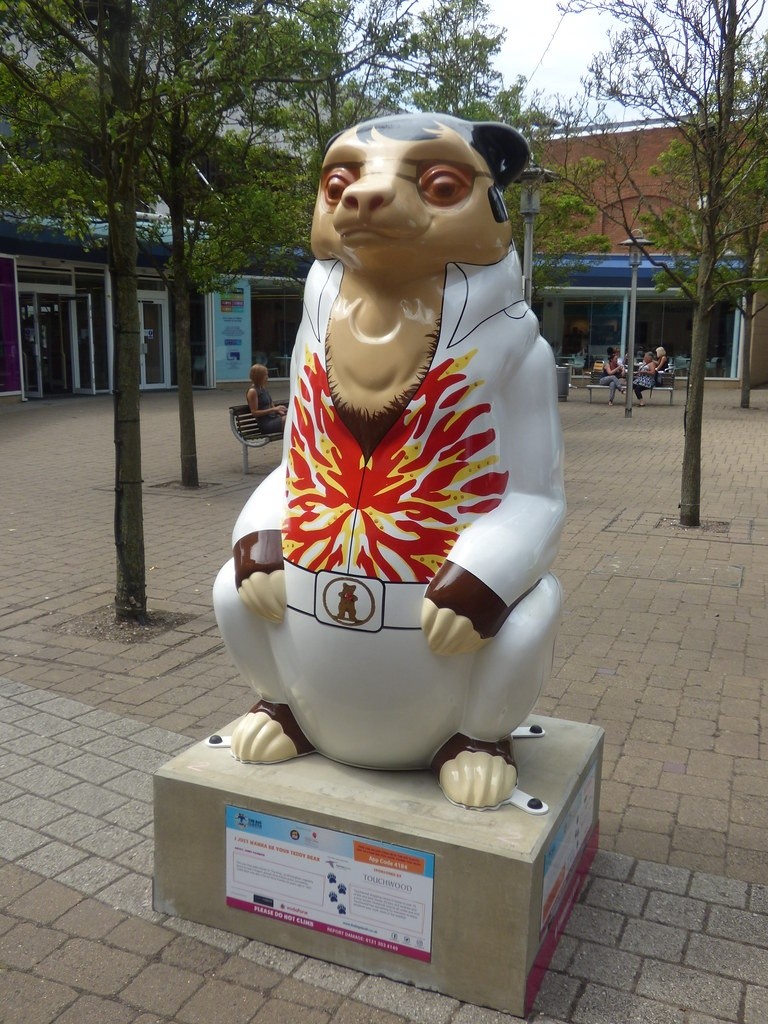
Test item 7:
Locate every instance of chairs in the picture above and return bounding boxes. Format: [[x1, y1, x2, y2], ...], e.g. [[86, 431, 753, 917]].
[[674, 356, 690, 377], [707, 356, 719, 377], [263, 357, 280, 377], [567, 356, 586, 375]]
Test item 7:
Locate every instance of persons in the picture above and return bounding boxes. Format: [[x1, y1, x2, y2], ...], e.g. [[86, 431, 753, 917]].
[[599, 354, 626, 406], [633, 352, 655, 407], [246, 364, 288, 435], [653, 347, 669, 373]]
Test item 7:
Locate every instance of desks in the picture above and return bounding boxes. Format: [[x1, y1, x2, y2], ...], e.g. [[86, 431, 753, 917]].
[[273, 356, 291, 377], [686, 358, 709, 377], [555, 356, 575, 375]]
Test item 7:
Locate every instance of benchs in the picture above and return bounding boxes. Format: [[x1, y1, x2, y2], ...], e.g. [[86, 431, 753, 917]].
[[584, 359, 677, 406], [228, 399, 289, 475]]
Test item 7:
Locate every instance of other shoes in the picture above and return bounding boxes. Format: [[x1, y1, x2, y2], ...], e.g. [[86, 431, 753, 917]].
[[621, 390, 626, 394], [636, 404, 645, 407], [609, 401, 613, 406]]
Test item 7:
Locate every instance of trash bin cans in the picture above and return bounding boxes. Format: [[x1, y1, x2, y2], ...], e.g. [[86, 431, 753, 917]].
[[557, 366, 570, 402]]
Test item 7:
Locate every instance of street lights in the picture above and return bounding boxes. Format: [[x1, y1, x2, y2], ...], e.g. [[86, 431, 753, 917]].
[[618, 230, 655, 419], [515, 154, 561, 309]]
